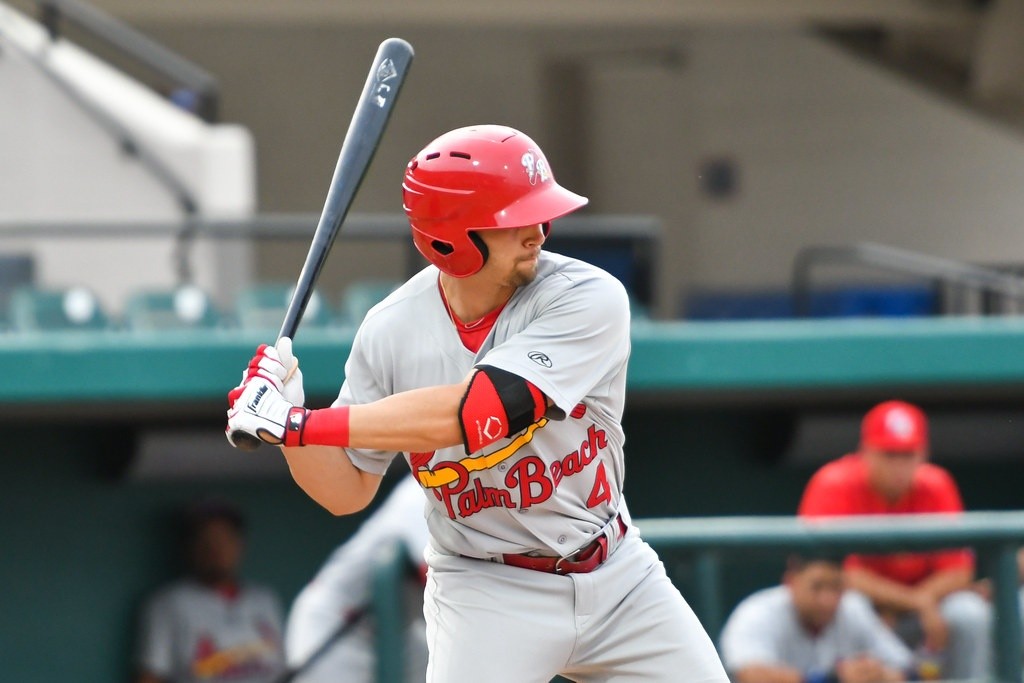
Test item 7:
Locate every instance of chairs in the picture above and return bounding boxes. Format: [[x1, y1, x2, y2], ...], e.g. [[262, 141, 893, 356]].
[[0, 277, 402, 332]]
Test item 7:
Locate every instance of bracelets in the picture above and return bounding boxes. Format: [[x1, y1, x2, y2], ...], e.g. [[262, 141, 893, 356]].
[[905, 668, 921, 683], [302, 406, 349, 447], [803, 668, 835, 683]]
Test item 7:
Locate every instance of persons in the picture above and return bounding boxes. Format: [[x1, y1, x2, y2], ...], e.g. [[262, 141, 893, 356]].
[[780, 400, 995, 683], [283, 469, 430, 683], [135, 506, 287, 683], [226, 125, 731, 683], [719, 545, 919, 683]]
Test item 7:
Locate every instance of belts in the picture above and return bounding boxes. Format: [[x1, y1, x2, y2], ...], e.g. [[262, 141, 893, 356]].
[[503, 514, 627, 574]]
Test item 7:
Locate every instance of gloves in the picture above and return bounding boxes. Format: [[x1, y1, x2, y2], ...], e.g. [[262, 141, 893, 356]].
[[225, 336, 311, 448]]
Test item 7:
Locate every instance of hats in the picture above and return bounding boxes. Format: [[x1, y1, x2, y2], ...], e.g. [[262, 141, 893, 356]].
[[860, 401, 927, 452]]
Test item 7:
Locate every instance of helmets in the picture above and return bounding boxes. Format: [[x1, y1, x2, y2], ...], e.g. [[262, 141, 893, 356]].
[[402, 124, 588, 278]]
